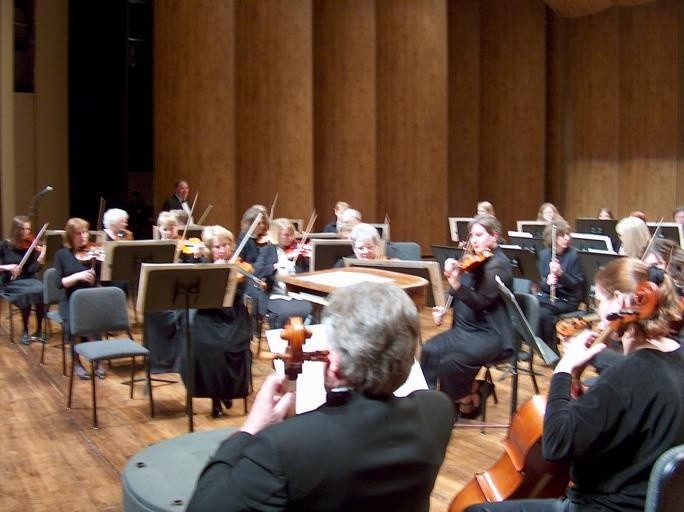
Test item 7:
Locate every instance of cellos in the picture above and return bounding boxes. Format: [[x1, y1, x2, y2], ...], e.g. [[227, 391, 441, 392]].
[[448, 281, 660, 512]]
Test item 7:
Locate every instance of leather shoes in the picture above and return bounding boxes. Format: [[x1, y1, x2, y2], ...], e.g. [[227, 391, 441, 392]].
[[460, 380, 492, 419]]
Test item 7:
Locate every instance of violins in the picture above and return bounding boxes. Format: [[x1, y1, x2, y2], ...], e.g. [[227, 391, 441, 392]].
[[19, 236, 45, 250], [232, 257, 267, 289], [74, 241, 105, 264], [285, 239, 311, 260], [444, 250, 493, 277]]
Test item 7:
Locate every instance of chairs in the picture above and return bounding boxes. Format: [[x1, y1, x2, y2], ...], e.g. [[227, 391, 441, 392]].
[[7, 242, 629, 380], [67, 286, 154, 429], [644, 444, 684, 511], [480, 292, 540, 423]]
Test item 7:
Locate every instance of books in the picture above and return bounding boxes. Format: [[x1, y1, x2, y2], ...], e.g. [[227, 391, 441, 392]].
[[265, 325, 429, 415]]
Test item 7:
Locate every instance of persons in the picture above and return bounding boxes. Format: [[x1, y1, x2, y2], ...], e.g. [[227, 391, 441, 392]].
[[158, 200, 400, 340], [185, 283, 454, 512], [95, 208, 134, 301], [126, 190, 148, 240], [55, 219, 105, 380], [163, 180, 195, 225], [0, 214, 51, 345], [419, 201, 683, 512]]
